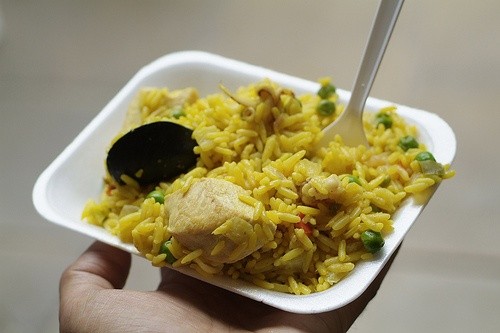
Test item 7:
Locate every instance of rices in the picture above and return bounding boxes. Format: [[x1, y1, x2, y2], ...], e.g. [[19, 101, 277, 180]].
[[85, 80, 454, 294]]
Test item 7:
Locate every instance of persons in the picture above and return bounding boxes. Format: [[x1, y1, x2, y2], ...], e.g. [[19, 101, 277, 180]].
[[58, 231, 405, 333]]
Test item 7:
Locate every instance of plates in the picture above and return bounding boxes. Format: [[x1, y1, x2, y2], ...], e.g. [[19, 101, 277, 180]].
[[30, 49, 459, 315]]
